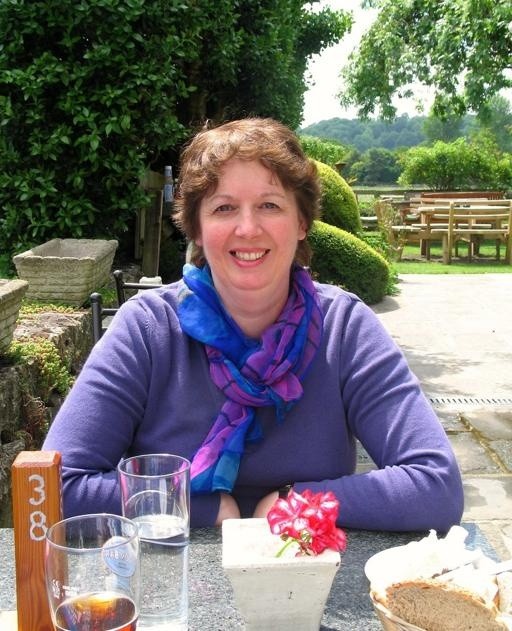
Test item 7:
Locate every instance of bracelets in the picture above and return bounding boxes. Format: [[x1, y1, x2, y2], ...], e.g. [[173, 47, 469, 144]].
[[277, 484, 294, 500]]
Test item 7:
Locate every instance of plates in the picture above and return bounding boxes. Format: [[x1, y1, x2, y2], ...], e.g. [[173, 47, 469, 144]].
[[365, 544, 498, 587]]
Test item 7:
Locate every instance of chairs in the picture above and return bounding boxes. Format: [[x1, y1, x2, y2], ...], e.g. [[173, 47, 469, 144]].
[[90, 292, 120, 347], [113, 269, 168, 307]]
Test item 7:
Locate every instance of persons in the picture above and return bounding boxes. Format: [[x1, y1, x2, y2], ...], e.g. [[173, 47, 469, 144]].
[[34, 114, 467, 537]]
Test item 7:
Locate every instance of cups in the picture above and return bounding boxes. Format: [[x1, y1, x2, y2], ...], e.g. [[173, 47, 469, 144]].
[[40, 513, 141, 631], [117, 452, 191, 631]]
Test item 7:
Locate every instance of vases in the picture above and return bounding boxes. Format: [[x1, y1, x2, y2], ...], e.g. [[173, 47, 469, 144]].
[[221, 518, 342, 631]]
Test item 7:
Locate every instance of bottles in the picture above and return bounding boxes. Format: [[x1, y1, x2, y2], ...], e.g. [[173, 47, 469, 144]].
[[162, 165, 175, 204]]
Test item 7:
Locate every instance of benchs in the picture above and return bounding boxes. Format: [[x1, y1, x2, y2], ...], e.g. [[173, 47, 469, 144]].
[[376, 189, 512, 265]]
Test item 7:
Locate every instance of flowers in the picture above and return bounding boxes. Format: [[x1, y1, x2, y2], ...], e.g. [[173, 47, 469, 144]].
[[264, 489, 349, 563]]
